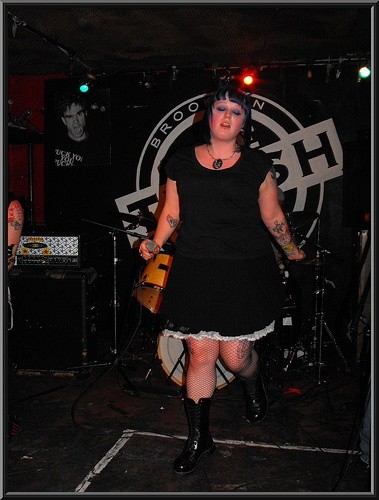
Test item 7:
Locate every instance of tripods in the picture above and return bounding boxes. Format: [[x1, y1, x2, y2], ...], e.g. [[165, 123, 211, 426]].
[[109, 270, 157, 368], [268, 251, 353, 383]]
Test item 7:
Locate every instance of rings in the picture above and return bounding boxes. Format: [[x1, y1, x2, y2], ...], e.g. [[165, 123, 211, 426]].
[[139, 252, 144, 256]]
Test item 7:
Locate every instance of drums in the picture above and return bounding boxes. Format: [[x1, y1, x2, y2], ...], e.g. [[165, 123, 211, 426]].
[[134, 251, 173, 314], [157, 330, 237, 392], [278, 262, 295, 310]]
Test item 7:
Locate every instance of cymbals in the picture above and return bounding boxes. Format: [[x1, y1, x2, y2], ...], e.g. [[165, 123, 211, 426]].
[[304, 259, 332, 266], [284, 210, 320, 223], [118, 212, 156, 229]]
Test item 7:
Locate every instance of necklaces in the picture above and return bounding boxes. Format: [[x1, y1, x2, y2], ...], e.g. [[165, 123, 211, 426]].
[[206, 144, 236, 169]]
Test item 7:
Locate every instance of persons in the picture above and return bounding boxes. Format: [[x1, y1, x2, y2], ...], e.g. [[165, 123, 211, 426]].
[[45, 95, 106, 209], [7, 192, 25, 269], [138, 87, 307, 474]]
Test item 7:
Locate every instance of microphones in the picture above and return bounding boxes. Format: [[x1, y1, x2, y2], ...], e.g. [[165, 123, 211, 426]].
[[115, 213, 156, 230]]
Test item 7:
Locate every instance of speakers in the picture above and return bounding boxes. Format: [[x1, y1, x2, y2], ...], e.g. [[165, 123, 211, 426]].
[[3, 268, 115, 370]]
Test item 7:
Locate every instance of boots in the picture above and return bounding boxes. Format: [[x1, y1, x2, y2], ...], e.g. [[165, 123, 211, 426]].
[[173, 397, 215, 475], [236, 363, 267, 424]]
